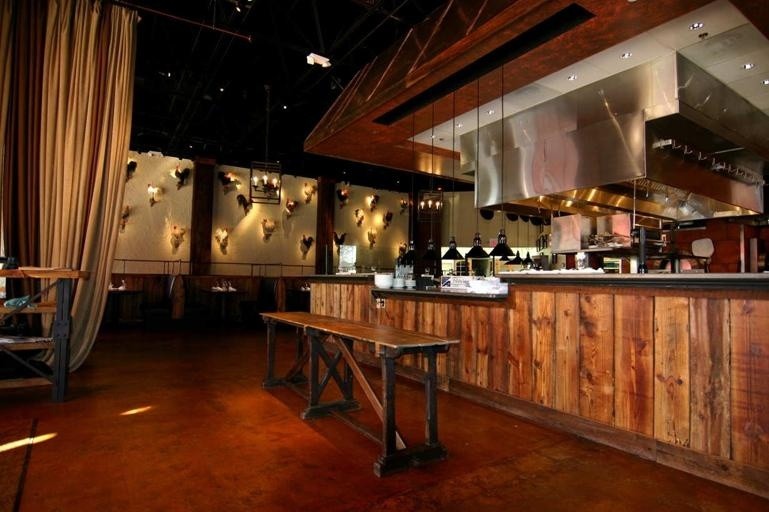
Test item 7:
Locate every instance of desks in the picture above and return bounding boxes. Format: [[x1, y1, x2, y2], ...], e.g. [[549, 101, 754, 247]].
[[261, 307, 462, 477], [108, 274, 310, 329]]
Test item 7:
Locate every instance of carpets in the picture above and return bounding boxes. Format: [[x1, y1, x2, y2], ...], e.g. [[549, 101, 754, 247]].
[[1, 416, 38, 512]]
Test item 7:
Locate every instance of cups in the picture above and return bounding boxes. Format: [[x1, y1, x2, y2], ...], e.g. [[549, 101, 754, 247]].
[[338, 246, 356, 273]]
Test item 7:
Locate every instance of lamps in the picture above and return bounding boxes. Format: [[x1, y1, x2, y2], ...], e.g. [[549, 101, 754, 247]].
[[253, 173, 279, 194], [397, 65, 534, 269]]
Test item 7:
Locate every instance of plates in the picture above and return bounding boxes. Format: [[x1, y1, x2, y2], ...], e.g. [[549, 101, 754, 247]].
[[587, 243, 624, 249], [374, 274, 416, 289], [500, 268, 605, 274]]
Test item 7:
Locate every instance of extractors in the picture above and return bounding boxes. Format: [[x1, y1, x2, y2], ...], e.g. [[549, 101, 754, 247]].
[[618, 175, 695, 203]]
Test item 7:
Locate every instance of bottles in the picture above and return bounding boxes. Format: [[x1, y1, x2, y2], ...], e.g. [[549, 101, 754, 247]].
[[575, 252, 588, 270], [637, 261, 648, 274]]
